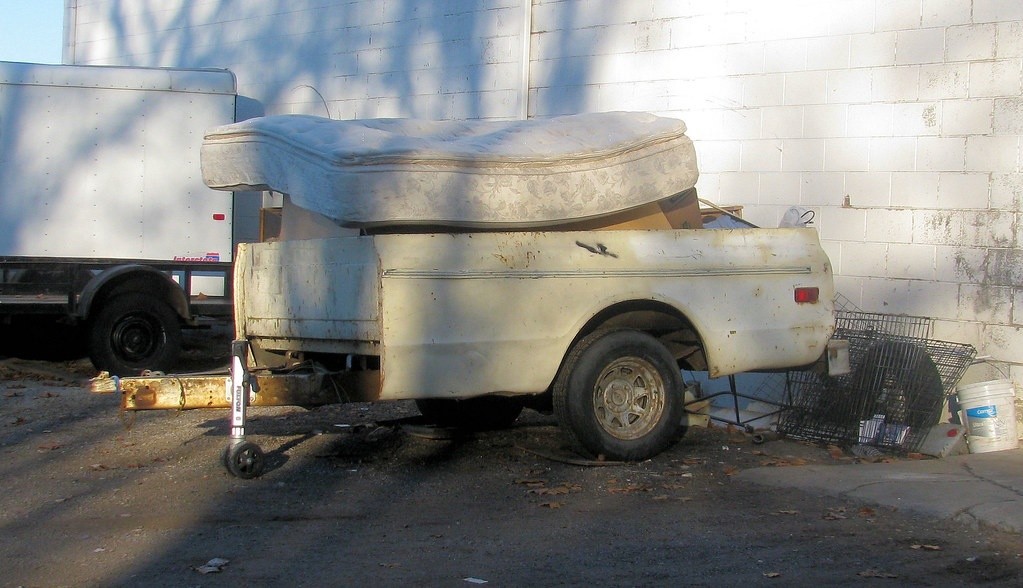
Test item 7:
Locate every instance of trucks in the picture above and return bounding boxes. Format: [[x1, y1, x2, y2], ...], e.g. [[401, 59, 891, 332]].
[[0, 59, 238, 377]]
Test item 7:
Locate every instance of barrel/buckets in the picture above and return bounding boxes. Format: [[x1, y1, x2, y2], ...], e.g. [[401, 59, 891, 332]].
[[954, 355, 1018, 454]]
